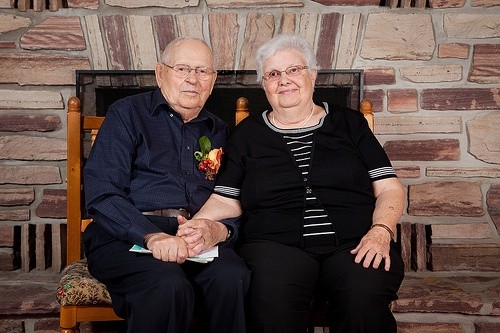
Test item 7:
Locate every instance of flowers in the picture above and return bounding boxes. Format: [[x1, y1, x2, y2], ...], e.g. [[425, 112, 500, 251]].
[[195, 136, 225, 182]]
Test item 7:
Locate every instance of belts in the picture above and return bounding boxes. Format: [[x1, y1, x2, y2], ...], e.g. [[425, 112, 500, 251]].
[[141, 208, 190, 218]]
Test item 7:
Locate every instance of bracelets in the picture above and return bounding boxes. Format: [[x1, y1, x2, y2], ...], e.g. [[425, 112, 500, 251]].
[[215, 220, 234, 246], [370, 223, 394, 241]]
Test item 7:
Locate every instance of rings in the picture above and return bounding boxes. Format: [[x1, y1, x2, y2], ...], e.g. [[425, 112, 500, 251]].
[[200, 236, 206, 243], [376, 253, 383, 257]]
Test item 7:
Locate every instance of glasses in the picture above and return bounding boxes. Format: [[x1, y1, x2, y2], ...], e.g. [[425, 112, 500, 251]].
[[262, 65, 310, 81], [162, 62, 215, 79]]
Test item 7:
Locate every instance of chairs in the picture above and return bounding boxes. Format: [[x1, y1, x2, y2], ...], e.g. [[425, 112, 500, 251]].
[[57, 97, 123, 333], [235, 97, 374, 135]]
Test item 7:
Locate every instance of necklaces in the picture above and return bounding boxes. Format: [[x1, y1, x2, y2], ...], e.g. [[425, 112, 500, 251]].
[[272, 102, 315, 129]]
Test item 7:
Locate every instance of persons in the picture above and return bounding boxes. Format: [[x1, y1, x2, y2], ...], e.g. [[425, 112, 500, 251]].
[[82, 36, 253, 333], [177, 32, 405, 333]]
